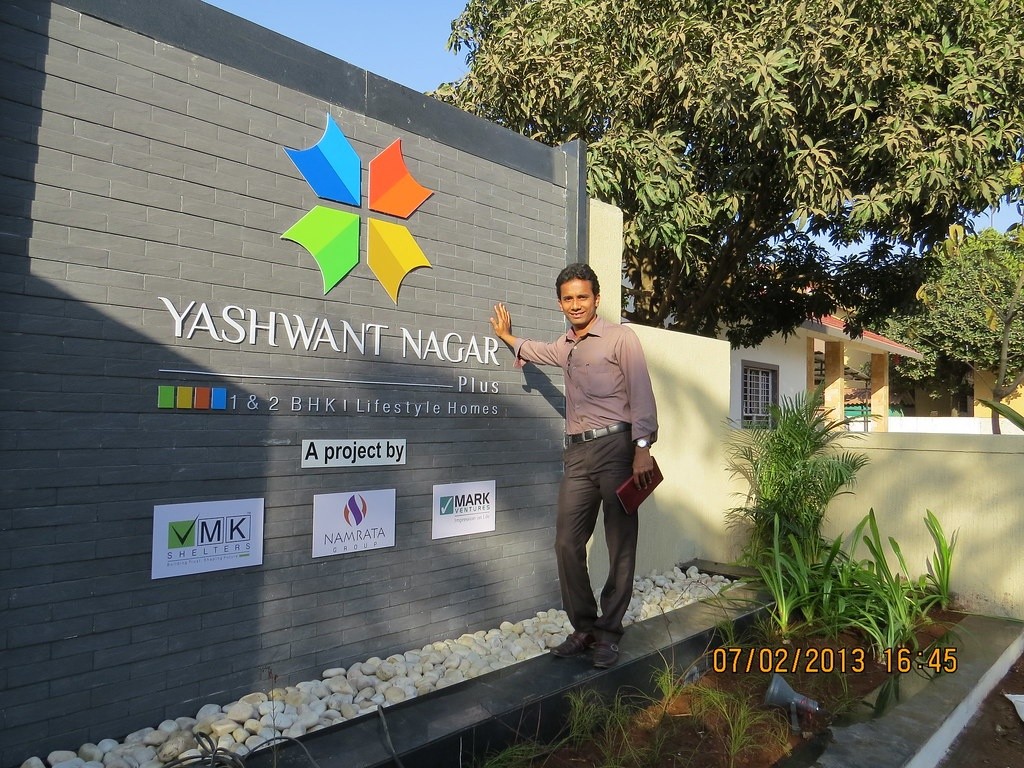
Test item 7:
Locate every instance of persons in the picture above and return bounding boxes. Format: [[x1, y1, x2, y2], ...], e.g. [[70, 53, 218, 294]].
[[490, 263, 659, 668]]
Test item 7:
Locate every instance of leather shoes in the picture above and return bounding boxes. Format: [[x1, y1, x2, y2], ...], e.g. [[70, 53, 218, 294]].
[[592, 642, 619, 667], [550, 630, 597, 657]]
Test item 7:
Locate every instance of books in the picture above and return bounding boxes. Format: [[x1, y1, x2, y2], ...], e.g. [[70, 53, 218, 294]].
[[616, 458, 664, 514]]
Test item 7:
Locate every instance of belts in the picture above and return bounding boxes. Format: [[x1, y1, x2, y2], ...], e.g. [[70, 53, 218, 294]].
[[567, 422, 632, 445]]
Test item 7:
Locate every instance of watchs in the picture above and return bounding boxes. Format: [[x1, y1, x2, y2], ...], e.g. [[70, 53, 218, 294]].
[[634, 438, 651, 449]]
